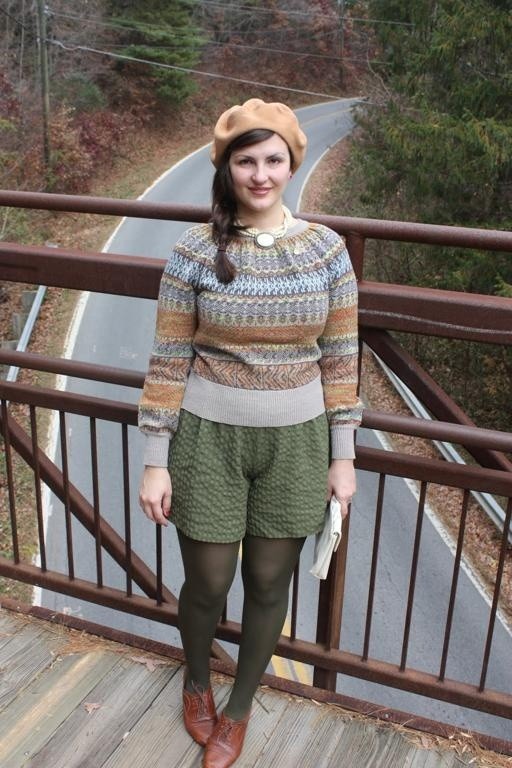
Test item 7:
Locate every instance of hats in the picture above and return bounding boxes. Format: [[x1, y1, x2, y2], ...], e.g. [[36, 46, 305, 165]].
[[209, 97, 307, 173]]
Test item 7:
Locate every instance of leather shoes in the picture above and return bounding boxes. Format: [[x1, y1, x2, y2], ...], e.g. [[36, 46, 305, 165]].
[[181, 666, 252, 767]]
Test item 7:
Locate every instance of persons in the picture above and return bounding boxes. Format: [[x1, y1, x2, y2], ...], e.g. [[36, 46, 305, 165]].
[[138, 96, 366, 766]]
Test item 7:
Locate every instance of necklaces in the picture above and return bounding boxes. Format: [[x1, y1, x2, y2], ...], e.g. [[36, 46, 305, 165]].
[[234, 205, 293, 249]]
